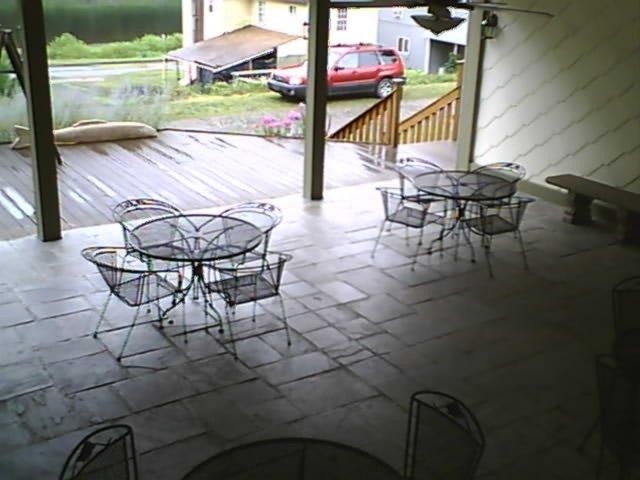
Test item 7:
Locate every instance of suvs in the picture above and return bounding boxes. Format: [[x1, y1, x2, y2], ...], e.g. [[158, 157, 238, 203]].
[[265, 43, 407, 102]]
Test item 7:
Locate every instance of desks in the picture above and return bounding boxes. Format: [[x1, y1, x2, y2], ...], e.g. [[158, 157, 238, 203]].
[[179, 438, 397, 480]]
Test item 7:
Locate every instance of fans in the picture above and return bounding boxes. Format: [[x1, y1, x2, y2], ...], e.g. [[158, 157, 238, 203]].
[[329, 0, 556, 21]]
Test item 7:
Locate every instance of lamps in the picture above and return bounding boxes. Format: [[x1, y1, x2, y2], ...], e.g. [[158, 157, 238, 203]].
[[482, 12, 496, 41], [411, 6, 465, 37]]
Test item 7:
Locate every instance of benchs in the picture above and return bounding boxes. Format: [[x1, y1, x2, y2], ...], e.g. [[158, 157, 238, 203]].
[[547, 173, 640, 244]]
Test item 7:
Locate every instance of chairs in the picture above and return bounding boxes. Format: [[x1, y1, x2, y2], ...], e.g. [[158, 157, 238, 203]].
[[369, 158, 535, 279], [57, 425, 138, 480], [615, 272, 638, 352], [402, 389, 486, 479], [81, 198, 291, 363], [574, 354, 639, 476]]
